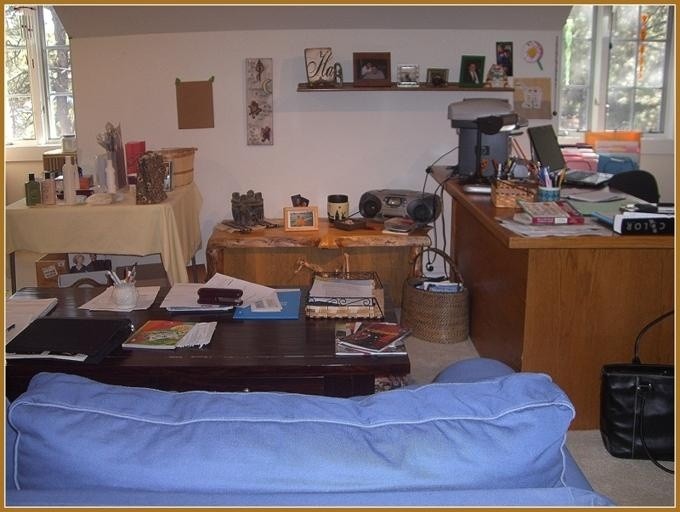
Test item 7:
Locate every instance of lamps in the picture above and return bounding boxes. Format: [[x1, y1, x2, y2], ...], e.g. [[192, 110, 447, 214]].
[[465, 114, 518, 193]]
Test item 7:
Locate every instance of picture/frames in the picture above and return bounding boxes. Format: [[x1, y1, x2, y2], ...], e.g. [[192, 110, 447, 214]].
[[513, 78, 551, 120], [243, 57, 273, 144], [527, 125, 567, 173], [176, 80, 214, 128], [426, 69, 449, 87], [496, 41, 513, 76], [458, 54, 484, 87], [283, 206, 318, 230], [304, 48, 337, 88], [518, 39, 547, 71], [352, 52, 390, 88], [396, 63, 418, 86], [162, 161, 172, 192]]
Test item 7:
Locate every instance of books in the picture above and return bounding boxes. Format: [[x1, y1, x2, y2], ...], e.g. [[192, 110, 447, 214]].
[[122, 320, 198, 349], [334, 321, 413, 355], [381, 216, 417, 235], [513, 199, 585, 225], [414, 277, 459, 293]]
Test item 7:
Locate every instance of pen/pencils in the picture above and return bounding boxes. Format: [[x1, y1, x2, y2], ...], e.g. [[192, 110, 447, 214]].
[[492, 158, 517, 180], [107, 266, 136, 284], [537, 165, 565, 188], [6, 323, 15, 332]]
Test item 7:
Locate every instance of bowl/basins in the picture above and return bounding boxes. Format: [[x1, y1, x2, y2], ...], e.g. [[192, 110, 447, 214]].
[[145, 147, 198, 189]]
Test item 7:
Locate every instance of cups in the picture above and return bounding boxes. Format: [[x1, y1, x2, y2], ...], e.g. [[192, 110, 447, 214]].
[[327, 194, 350, 224]]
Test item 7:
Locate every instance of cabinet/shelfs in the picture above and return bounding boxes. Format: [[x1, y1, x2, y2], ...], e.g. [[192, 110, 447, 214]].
[[207, 218, 431, 308], [297, 83, 515, 91]]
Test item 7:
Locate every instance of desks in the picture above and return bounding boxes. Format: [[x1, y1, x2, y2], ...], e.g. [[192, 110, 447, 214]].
[[426, 164, 674, 429], [4, 183, 200, 295]]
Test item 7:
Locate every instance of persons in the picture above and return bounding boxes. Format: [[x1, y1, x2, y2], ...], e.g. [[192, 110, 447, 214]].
[[70, 255, 88, 273], [464, 63, 479, 83], [359, 61, 371, 78], [86, 253, 105, 272], [363, 65, 385, 79]]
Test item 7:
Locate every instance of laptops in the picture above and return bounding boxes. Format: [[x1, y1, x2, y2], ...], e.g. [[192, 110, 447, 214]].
[[527, 125, 614, 189]]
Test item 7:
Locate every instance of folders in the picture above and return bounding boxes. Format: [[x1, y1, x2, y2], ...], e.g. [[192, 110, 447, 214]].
[[7, 317, 131, 374]]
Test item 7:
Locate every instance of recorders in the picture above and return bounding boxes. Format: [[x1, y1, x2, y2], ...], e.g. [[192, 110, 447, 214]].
[[358, 189, 442, 223]]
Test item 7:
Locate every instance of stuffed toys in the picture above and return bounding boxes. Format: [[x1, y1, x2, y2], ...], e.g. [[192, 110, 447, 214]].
[[483, 64, 511, 87]]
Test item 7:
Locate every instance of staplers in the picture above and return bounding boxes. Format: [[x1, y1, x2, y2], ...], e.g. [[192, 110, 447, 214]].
[[197, 287, 243, 306]]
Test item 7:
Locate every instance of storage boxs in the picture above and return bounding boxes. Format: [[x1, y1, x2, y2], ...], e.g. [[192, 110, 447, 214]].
[[117, 262, 207, 287], [43, 149, 76, 180], [149, 149, 196, 187], [36, 255, 67, 287], [587, 131, 642, 172]]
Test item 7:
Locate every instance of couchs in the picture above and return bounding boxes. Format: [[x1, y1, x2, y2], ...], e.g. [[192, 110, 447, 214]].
[[5, 359, 603, 510]]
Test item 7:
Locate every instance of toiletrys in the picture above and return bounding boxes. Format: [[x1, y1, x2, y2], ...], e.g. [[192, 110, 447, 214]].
[[62, 156, 77, 202], [41, 173, 56, 205], [25, 174, 40, 206], [105, 160, 116, 193]]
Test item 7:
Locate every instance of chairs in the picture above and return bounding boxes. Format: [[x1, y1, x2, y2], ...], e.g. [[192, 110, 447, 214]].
[[609, 170, 659, 202]]
